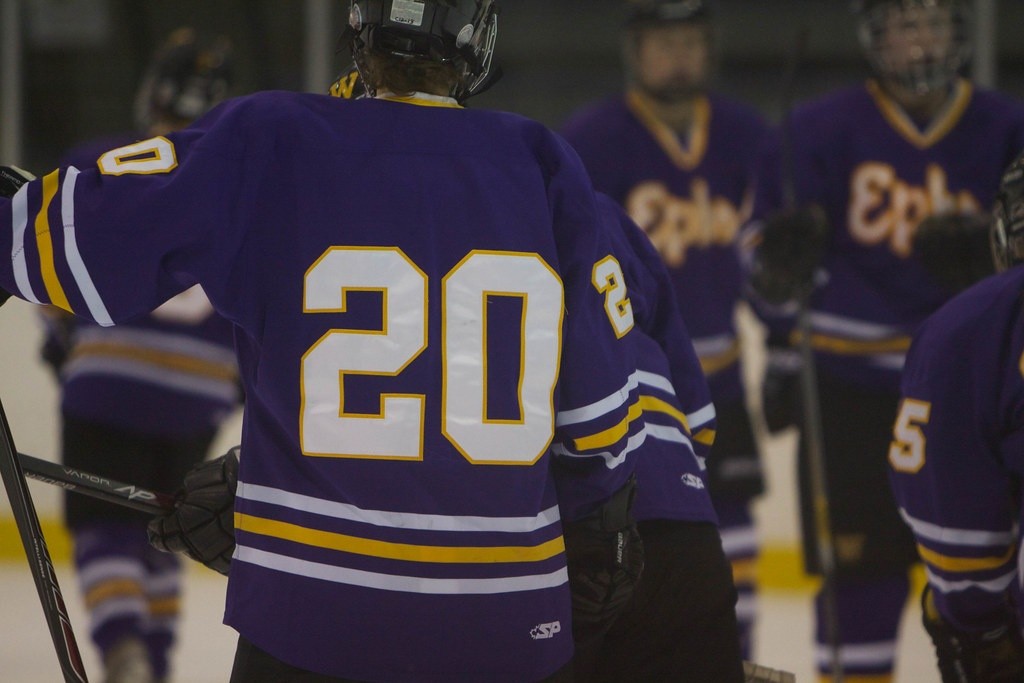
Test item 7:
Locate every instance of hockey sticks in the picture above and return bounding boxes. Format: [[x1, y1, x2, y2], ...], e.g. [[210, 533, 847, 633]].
[[797, 301, 844, 682], [17, 451, 175, 518], [1, 399, 89, 683]]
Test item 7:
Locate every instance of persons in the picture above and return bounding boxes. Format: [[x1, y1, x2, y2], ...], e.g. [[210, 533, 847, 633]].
[[888, 152, 1024, 683], [0, 0, 648, 683], [332, 64, 745, 683], [39, 28, 242, 683], [554, 0, 774, 683], [760, 0, 1024, 683]]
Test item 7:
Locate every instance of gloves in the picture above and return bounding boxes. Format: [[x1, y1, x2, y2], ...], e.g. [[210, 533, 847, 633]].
[[142, 446, 247, 581], [565, 473, 651, 652]]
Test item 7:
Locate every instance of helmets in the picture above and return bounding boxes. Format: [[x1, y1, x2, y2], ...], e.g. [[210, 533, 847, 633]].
[[985, 149, 1023, 277], [851, 0, 985, 93], [327, 1, 508, 104], [131, 25, 278, 137], [623, 0, 719, 99]]
